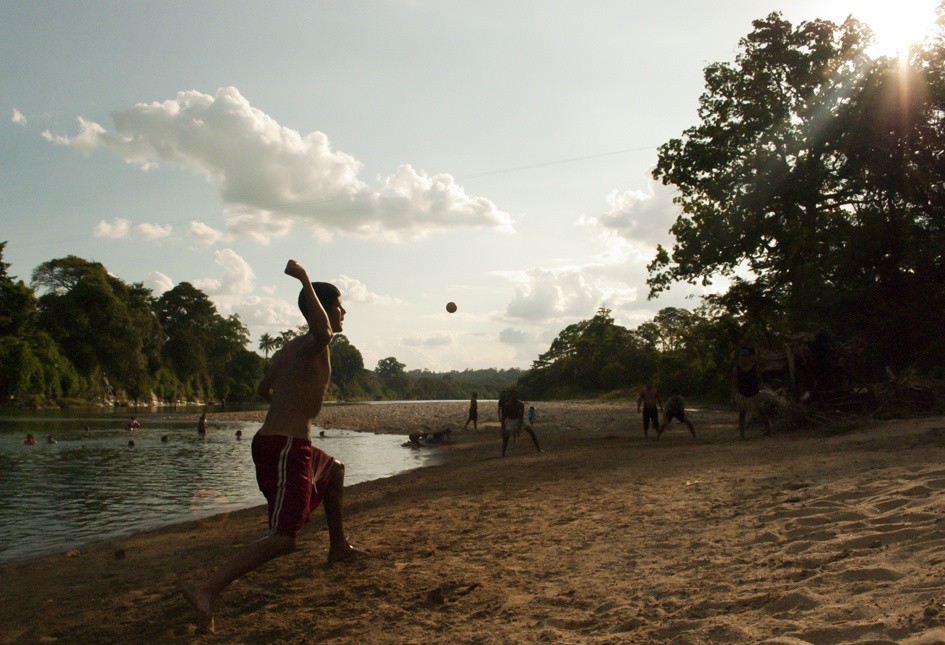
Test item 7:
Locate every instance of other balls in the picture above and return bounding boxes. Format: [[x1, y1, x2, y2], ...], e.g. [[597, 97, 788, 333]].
[[446, 303, 457, 313]]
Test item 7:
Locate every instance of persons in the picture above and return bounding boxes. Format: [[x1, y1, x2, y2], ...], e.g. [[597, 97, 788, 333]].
[[729, 348, 773, 437], [496, 390, 545, 456], [221, 397, 225, 411], [128, 416, 140, 431], [636, 380, 664, 438], [527, 406, 535, 427], [320, 431, 325, 437], [23, 433, 38, 446], [84, 425, 90, 432], [429, 428, 451, 443], [47, 435, 58, 444], [161, 434, 170, 443], [127, 440, 135, 448], [235, 430, 242, 439], [656, 389, 697, 440], [401, 432, 428, 446], [178, 260, 368, 624], [463, 394, 478, 429], [198, 414, 208, 440]]
